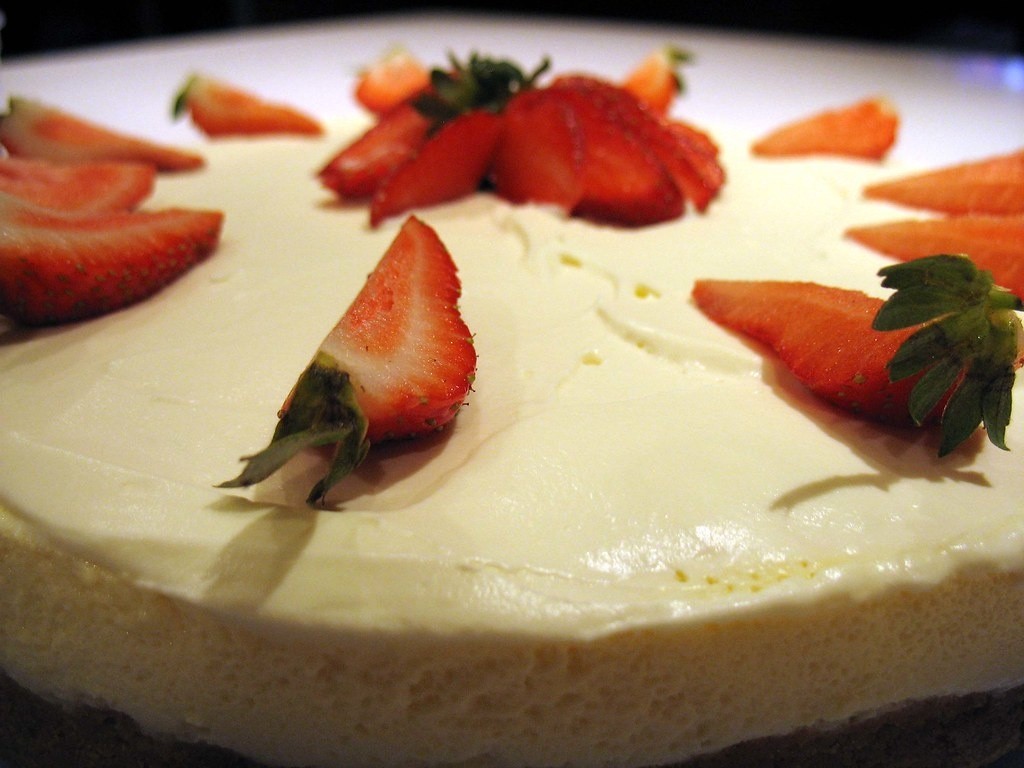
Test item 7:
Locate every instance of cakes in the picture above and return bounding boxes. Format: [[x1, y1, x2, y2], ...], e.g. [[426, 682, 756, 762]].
[[0, 44, 1024, 768]]
